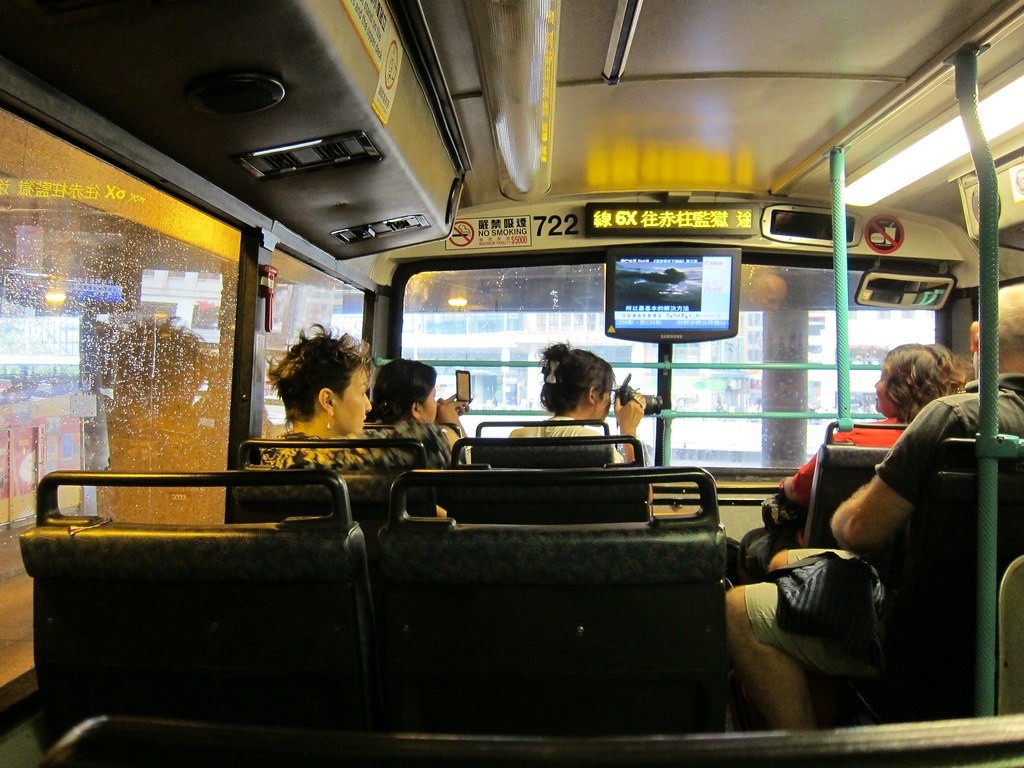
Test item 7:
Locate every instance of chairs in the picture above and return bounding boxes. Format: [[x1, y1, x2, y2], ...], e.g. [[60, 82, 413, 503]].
[[450, 436, 649, 526], [800, 425, 911, 549], [473, 420, 614, 467], [226, 435, 435, 525], [19, 472, 372, 729], [374, 467, 732, 734]]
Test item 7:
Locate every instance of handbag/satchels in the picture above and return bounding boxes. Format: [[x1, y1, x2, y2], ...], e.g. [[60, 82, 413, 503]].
[[767, 547, 886, 644]]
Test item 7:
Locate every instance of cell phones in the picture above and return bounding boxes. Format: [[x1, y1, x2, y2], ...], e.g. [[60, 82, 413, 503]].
[[456, 370, 471, 402]]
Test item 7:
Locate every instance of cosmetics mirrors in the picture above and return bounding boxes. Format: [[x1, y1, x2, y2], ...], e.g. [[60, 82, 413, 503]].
[[447, 369, 472, 411]]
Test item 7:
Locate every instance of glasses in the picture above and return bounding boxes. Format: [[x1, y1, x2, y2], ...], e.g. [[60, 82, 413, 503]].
[[330, 333, 360, 367]]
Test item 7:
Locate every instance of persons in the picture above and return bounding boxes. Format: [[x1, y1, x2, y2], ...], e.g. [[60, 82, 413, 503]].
[[261, 321, 474, 479], [507, 343, 653, 505], [724, 280, 1024, 729]]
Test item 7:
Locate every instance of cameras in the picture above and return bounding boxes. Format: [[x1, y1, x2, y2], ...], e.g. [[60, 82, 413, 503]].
[[616, 373, 663, 416]]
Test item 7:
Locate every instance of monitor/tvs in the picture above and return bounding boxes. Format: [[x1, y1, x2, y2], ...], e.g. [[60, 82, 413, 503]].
[[605, 248, 742, 344]]
